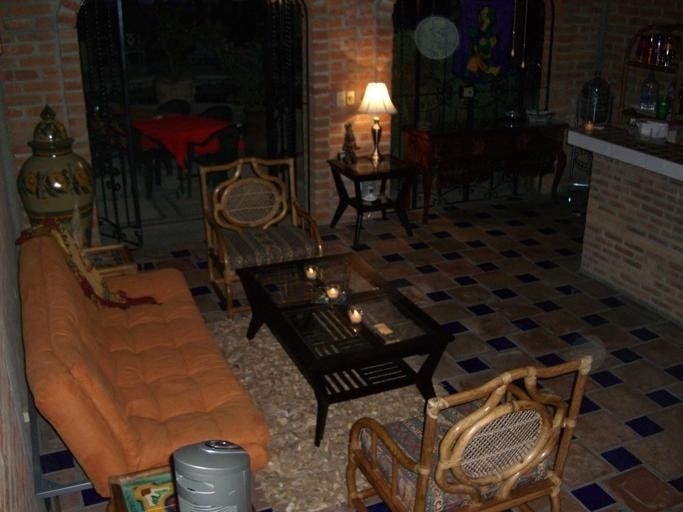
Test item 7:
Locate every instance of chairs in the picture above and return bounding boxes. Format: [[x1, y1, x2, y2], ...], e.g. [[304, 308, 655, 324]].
[[94, 98, 262, 200], [198, 155, 325, 322], [345, 352, 596, 512]]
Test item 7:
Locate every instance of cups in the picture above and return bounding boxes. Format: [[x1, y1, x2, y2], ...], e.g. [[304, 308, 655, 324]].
[[505, 109, 520, 128], [304, 265, 319, 280], [325, 284, 341, 300], [628, 117, 683, 146], [346, 307, 364, 325]]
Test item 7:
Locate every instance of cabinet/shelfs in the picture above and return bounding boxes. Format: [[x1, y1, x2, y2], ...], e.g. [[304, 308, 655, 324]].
[[618, 22, 682, 140]]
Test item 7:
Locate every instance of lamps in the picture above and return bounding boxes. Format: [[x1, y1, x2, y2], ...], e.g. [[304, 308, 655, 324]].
[[357, 81, 401, 164]]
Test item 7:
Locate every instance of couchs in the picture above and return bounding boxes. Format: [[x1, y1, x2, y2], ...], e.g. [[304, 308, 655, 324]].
[[13, 218, 271, 504]]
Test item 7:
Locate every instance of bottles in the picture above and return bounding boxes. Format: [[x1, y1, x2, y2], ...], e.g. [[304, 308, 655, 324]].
[[639, 71, 683, 123], [637, 30, 676, 68], [573, 70, 613, 173], [15, 105, 95, 252]]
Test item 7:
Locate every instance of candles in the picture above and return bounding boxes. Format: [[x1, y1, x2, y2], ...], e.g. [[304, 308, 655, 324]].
[[350, 309, 361, 324], [306, 267, 316, 280], [327, 286, 338, 299]]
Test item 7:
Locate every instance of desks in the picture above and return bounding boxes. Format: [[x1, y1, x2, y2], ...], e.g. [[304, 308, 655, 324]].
[[324, 153, 419, 248], [82, 243, 137, 278], [105, 462, 257, 511], [500, 122, 568, 206]]
